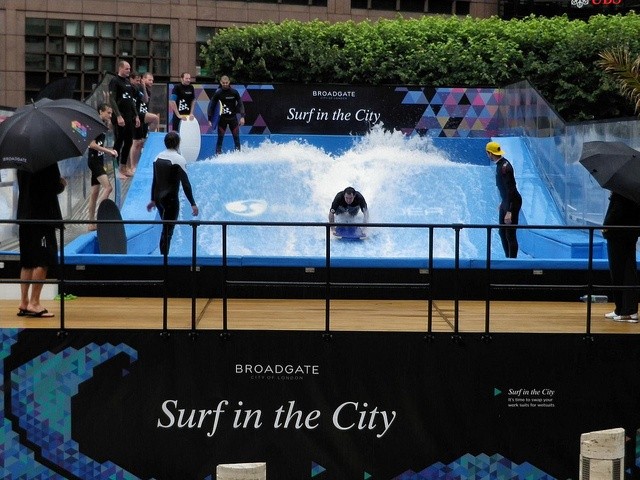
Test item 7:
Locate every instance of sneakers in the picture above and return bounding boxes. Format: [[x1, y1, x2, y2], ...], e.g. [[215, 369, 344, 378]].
[[605, 312, 617, 318], [613, 313, 639, 323]]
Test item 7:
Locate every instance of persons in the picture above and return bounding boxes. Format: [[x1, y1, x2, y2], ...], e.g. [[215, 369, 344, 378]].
[[85, 103, 118, 232], [168, 72, 196, 131], [207, 75, 245, 154], [147, 131, 198, 255], [127, 71, 148, 105], [108, 60, 142, 179], [16, 161, 67, 317], [601, 191, 640, 323], [328, 187, 368, 236], [129, 73, 159, 170], [486, 142, 523, 257]]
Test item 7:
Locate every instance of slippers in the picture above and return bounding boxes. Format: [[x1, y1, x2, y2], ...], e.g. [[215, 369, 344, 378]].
[[26, 307, 54, 317], [17, 306, 27, 317]]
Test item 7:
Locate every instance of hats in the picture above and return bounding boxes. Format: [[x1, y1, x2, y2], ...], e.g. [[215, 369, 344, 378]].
[[486, 141, 505, 156]]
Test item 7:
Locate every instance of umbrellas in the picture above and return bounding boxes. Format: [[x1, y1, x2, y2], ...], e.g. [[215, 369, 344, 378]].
[[0, 97, 110, 171], [579, 141, 640, 204]]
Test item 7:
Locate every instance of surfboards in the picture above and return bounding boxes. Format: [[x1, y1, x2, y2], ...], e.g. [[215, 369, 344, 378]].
[[178, 115, 202, 163], [112, 155, 121, 208], [210, 100, 223, 131], [97, 199, 128, 253], [336, 232, 361, 239]]
